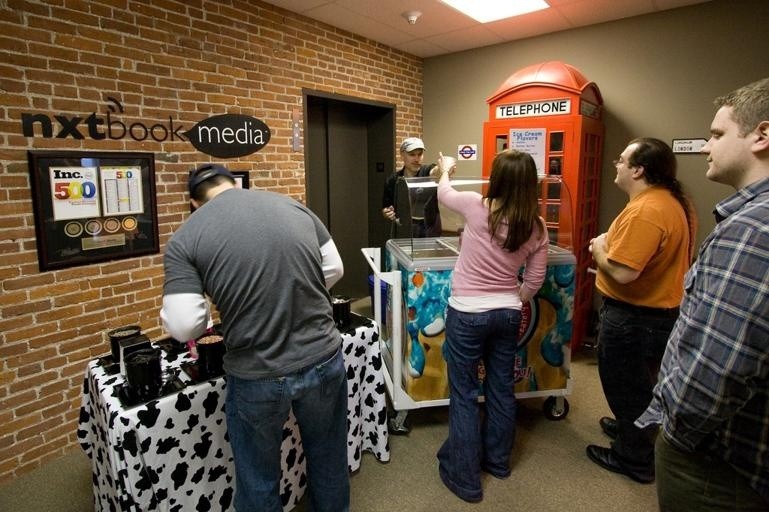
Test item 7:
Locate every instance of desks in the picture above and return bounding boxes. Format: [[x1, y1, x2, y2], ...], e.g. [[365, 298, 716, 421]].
[[73, 294, 396, 511]]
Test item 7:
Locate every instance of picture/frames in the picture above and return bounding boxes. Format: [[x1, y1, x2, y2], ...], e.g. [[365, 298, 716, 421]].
[[21, 144, 164, 271]]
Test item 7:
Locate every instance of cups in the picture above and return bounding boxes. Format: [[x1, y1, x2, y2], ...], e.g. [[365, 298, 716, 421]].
[[332, 295, 350, 324], [195, 333, 225, 380], [443, 155, 457, 177], [107, 326, 141, 363], [124, 349, 163, 401]]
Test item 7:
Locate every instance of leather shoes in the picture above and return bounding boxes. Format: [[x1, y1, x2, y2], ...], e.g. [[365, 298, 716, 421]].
[[586, 444, 655, 483], [600, 416, 618, 438]]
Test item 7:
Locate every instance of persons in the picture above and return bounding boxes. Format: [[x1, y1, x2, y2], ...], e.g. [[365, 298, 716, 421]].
[[586, 136, 697, 485], [382, 137, 456, 239], [634, 77, 769, 512], [159, 162, 350, 512], [436, 148, 550, 503]]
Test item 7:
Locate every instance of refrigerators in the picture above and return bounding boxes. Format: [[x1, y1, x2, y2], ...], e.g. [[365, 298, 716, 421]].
[[386, 237, 575, 407]]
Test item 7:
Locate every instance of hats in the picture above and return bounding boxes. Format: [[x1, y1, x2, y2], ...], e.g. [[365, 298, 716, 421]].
[[188, 165, 236, 214], [400, 137, 426, 153]]
